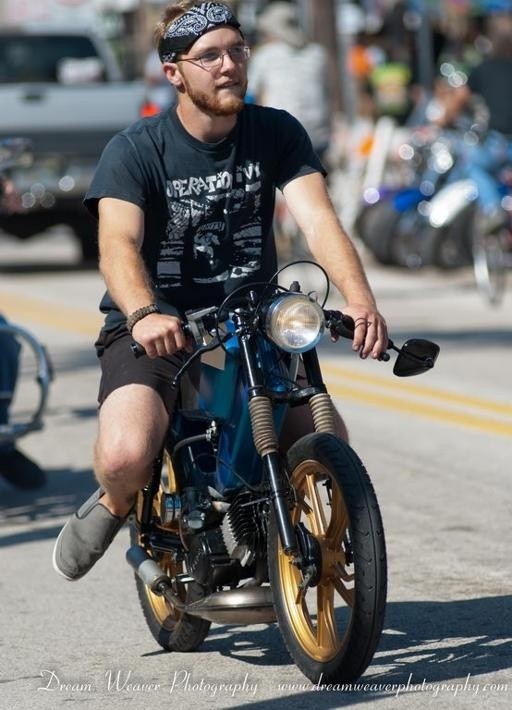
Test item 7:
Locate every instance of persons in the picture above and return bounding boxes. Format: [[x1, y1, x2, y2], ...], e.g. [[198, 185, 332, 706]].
[[49, 1, 388, 584], [141, 1, 511, 271]]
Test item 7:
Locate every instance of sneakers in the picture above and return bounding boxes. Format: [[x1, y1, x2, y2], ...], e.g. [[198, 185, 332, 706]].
[[52, 484, 134, 581], [0, 444, 44, 491]]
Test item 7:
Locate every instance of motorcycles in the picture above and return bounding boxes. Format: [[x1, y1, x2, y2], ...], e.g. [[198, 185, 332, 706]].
[[118, 256, 449, 694], [0, 311, 58, 455], [364, 53, 511, 317]]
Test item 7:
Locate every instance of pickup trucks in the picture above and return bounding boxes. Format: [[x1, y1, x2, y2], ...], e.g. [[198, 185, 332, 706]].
[[1, 19, 178, 270]]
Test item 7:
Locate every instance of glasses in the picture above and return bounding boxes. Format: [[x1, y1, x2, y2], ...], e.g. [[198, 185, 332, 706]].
[[176, 46, 251, 69]]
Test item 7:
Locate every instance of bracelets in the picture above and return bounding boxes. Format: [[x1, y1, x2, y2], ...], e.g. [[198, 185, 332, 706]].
[[125, 303, 162, 335]]
